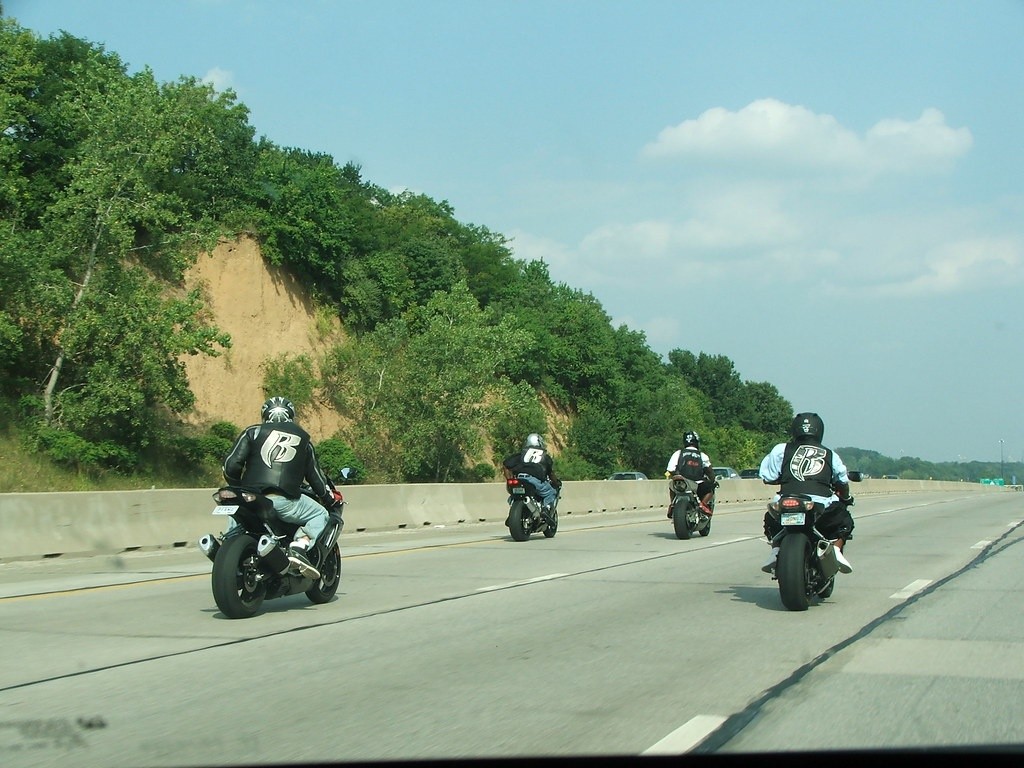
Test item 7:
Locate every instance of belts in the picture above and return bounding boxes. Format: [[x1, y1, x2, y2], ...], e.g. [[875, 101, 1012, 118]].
[[516, 474, 530, 478]]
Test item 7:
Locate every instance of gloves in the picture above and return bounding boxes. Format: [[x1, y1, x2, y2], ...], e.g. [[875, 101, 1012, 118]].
[[323, 485, 344, 508]]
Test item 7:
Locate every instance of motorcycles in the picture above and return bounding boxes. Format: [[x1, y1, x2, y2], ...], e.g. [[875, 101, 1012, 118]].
[[199, 466, 359, 618], [666, 474, 720, 539], [763, 468, 864, 611], [505, 477, 562, 541]]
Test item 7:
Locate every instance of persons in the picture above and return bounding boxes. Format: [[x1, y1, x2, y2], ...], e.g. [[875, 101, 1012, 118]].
[[502, 433, 562, 526], [223, 397, 342, 579], [666, 431, 717, 518], [759, 412, 855, 574]]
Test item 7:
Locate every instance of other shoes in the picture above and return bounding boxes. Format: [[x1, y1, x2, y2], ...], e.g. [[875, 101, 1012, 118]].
[[540, 507, 556, 526]]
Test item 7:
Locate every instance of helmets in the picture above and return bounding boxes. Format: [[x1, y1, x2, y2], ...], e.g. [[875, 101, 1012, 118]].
[[683, 431, 700, 448], [792, 412, 824, 443], [260, 397, 295, 422], [526, 434, 544, 448]]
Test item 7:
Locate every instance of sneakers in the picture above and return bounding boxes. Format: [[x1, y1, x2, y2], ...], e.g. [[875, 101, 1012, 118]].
[[288, 541, 320, 579], [699, 501, 713, 516], [667, 505, 674, 518], [833, 546, 852, 574], [761, 547, 780, 574]]
